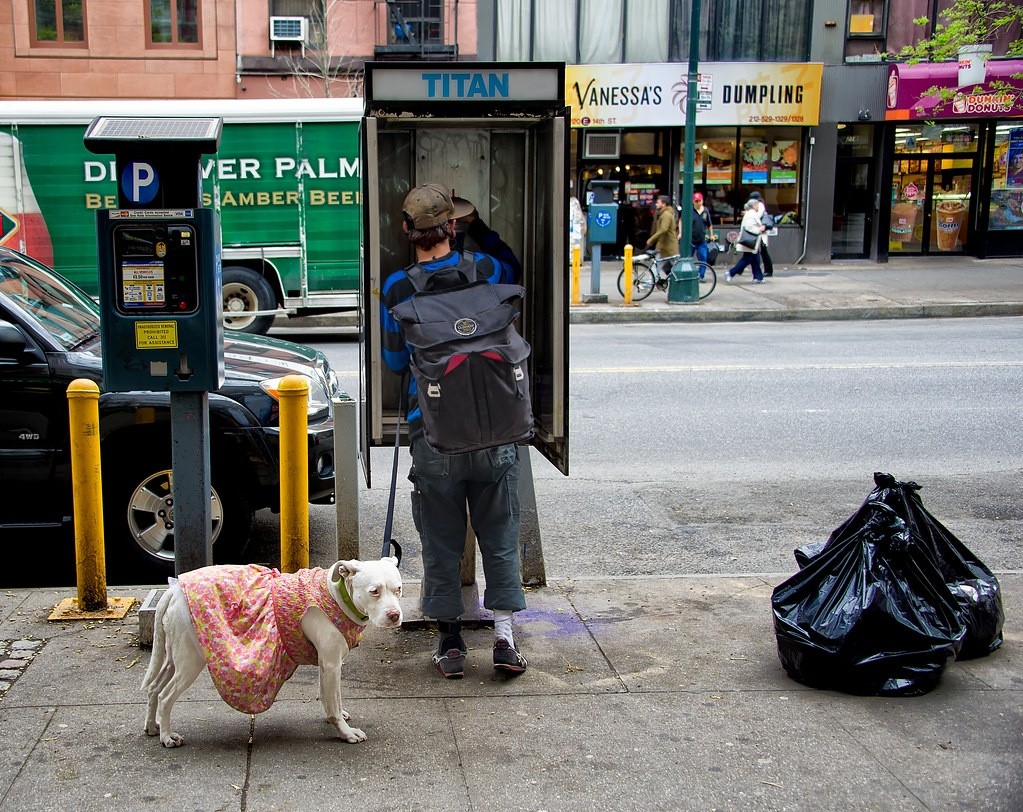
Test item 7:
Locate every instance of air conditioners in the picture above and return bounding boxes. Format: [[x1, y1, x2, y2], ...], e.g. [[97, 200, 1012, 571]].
[[585, 134, 620, 158], [270, 17, 305, 40]]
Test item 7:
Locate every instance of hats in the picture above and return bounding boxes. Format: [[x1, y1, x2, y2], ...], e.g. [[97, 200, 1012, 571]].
[[750, 192, 760, 201], [693, 192, 703, 202], [403, 182, 474, 230]]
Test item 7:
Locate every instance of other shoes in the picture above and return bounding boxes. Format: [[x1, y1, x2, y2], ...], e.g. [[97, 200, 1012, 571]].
[[752, 279, 765, 284], [699, 277, 706, 282], [726, 270, 732, 281], [762, 272, 772, 276]]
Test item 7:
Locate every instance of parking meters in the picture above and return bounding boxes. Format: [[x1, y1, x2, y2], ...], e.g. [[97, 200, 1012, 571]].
[[83, 113, 227, 575]]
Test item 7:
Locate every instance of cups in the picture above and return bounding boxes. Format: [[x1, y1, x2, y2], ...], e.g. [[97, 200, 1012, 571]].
[[937, 204, 966, 251], [890, 203, 917, 243], [959, 44, 992, 87]]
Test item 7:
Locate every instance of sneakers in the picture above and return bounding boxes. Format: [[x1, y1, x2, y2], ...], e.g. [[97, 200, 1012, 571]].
[[492, 637, 529, 671], [431, 648, 468, 677]]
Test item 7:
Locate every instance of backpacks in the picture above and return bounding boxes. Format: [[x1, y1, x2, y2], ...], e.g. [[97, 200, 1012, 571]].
[[388, 250, 537, 456]]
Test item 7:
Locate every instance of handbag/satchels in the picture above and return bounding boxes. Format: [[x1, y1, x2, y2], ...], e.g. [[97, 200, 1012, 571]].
[[740, 227, 759, 249], [706, 240, 719, 265], [761, 214, 774, 229]]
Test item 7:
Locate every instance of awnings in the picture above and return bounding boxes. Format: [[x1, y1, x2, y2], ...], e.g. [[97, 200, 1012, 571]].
[[885, 60, 1023, 120]]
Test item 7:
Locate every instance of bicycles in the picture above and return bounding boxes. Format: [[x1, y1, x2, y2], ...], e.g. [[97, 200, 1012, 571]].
[[617, 250, 716, 301]]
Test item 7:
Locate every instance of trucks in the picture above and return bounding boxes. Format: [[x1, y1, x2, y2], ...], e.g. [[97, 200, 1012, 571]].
[[0, 97, 367, 337]]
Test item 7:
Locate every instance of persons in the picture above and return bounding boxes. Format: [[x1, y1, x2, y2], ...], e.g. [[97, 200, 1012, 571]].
[[383, 183, 529, 679], [647, 196, 679, 277], [725, 191, 772, 283], [677, 192, 714, 283]]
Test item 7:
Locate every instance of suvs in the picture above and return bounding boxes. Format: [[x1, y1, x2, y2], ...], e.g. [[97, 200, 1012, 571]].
[[0, 244, 350, 586]]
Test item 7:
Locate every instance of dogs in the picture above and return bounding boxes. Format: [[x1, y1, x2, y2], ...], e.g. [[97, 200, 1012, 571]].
[[139, 556, 403, 749]]
